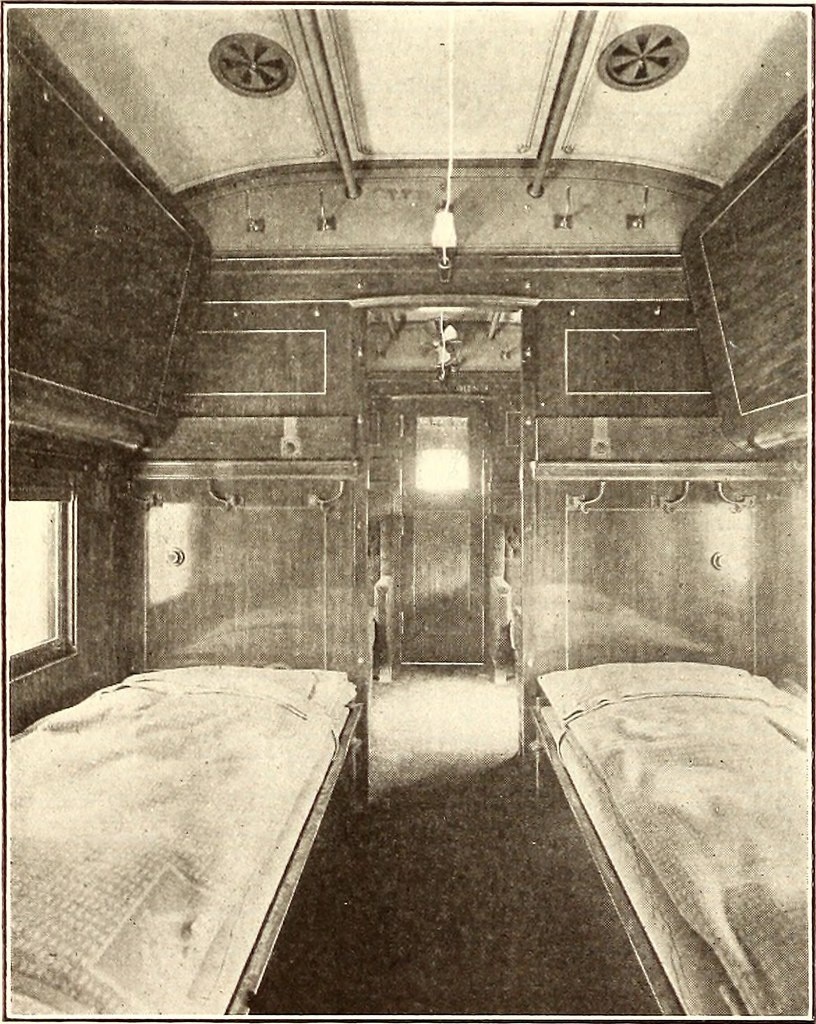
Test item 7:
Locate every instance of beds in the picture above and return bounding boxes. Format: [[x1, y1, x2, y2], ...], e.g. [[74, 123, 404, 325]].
[[5, 661, 366, 1018], [529, 665, 811, 1017]]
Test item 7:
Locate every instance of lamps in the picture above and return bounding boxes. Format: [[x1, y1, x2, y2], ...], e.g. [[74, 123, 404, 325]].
[[432, 208, 459, 284]]
